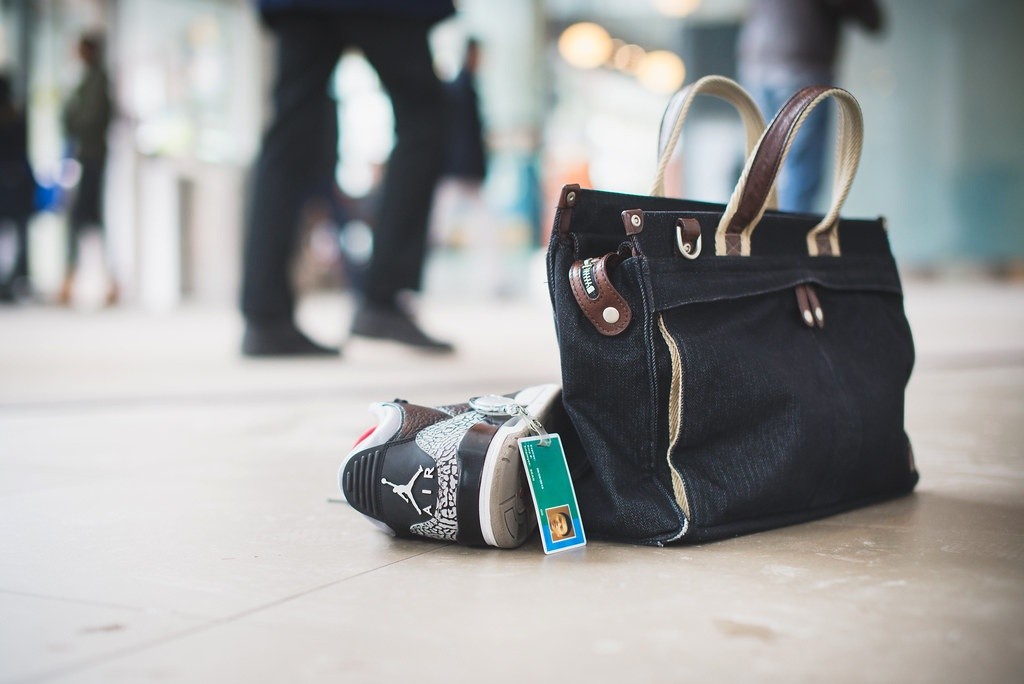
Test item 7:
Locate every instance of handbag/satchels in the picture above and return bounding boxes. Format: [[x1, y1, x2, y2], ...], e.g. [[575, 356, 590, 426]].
[[546, 75, 919, 546]]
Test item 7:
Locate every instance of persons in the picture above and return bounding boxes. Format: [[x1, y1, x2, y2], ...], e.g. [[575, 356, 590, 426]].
[[443, 35, 487, 193], [40, 28, 123, 310], [243, 0, 453, 361], [547, 511, 572, 539], [1, 71, 37, 303], [734, 0, 885, 210]]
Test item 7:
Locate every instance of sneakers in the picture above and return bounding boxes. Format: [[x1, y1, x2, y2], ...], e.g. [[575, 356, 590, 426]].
[[340, 384, 562, 547]]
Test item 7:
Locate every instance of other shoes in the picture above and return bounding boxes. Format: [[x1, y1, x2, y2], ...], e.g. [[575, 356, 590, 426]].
[[0, 279, 15, 305], [353, 306, 453, 353], [105, 286, 119, 305], [10, 266, 28, 281], [244, 319, 338, 357], [56, 277, 74, 301]]
